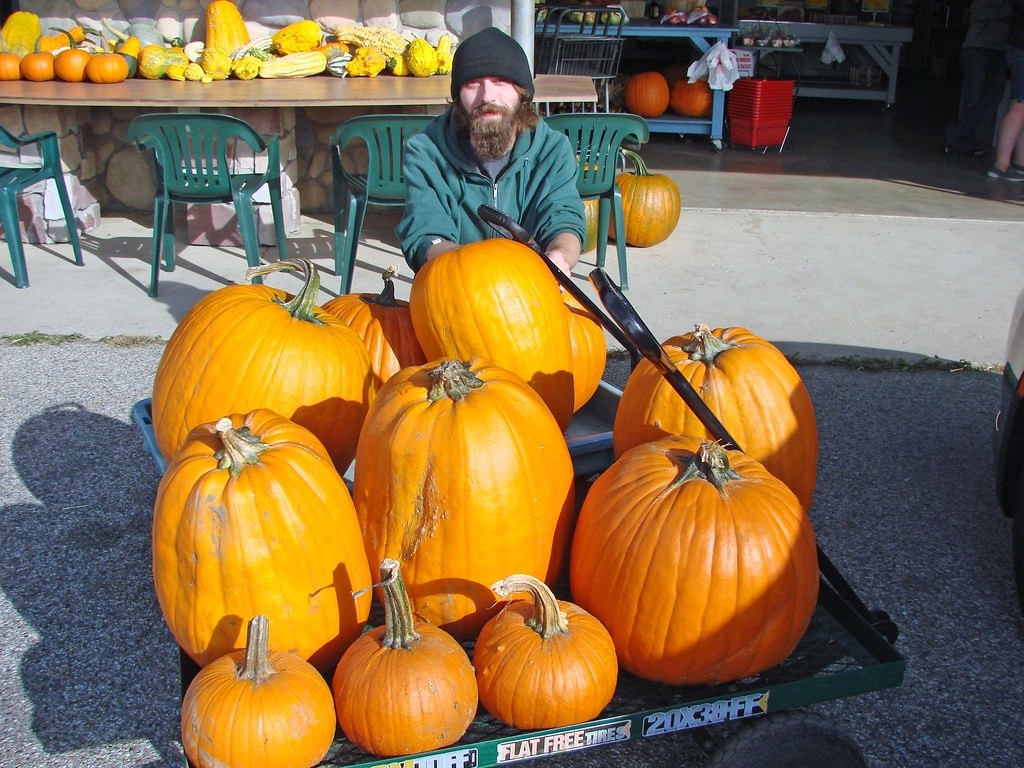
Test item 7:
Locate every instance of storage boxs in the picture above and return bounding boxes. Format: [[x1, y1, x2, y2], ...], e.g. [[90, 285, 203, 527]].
[[727, 74, 794, 149]]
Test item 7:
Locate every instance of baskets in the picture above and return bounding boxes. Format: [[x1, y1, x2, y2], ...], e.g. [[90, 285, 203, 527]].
[[727, 77, 797, 150]]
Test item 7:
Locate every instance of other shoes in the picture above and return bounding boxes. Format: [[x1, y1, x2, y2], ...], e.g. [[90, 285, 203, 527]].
[[988, 161, 1024, 181]]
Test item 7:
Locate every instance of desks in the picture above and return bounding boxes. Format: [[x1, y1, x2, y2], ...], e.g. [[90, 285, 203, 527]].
[[0, 75, 598, 107], [737, 45, 803, 102], [534, 19, 741, 154], [738, 19, 913, 108]]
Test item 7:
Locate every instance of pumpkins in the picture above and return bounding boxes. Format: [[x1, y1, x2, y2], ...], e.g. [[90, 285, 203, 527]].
[[0, 0, 455, 84], [626, 71, 713, 118], [150, 238, 818, 768], [579, 148, 681, 253]]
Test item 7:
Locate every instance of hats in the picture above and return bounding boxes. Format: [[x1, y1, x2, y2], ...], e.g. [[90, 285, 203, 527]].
[[449, 27, 534, 102]]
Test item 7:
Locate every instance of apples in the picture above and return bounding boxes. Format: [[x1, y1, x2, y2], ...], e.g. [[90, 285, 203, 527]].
[[663, 6, 718, 24], [569, 10, 627, 26]]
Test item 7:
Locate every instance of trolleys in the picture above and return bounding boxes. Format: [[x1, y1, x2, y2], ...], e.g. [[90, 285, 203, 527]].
[[131, 203, 910, 768], [534, 4, 628, 160]]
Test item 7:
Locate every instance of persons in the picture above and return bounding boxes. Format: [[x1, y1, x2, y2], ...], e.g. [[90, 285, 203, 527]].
[[986, 0, 1024, 181], [942, 0, 1013, 160], [395, 26, 587, 294]]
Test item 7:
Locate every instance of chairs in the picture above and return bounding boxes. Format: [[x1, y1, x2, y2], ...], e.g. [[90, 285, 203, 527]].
[[330, 114, 440, 298], [127, 113, 290, 300], [0, 125, 86, 289], [544, 113, 650, 291]]
